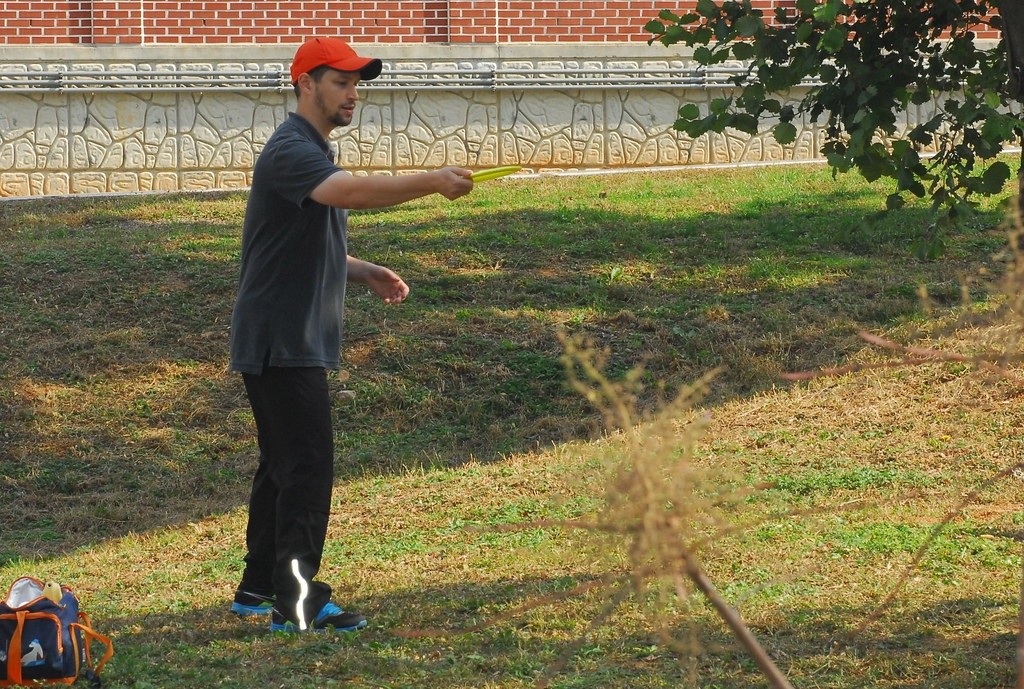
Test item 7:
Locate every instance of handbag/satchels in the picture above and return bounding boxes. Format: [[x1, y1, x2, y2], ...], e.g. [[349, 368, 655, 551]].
[[0, 575, 113, 688]]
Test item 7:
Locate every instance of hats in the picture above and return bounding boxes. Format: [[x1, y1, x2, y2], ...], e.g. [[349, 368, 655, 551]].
[[291, 38, 382, 87]]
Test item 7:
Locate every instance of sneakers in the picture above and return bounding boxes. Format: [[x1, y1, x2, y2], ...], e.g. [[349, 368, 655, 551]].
[[270, 600, 368, 634], [232, 589, 276, 615]]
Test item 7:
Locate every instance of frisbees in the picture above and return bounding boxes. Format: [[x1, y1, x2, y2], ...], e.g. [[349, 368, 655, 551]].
[[466, 166, 523, 182]]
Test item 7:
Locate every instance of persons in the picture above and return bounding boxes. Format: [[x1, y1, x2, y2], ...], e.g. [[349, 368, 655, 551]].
[[228, 36, 475, 639]]
